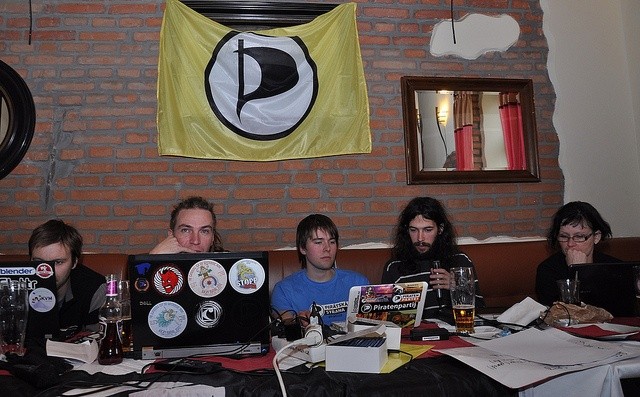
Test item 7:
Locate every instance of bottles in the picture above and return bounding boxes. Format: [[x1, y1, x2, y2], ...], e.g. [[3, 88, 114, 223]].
[[97, 273, 124, 364]]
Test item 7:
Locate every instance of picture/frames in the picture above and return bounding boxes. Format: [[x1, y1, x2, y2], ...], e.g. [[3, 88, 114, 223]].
[[0, 61, 37, 182], [400, 71, 541, 187]]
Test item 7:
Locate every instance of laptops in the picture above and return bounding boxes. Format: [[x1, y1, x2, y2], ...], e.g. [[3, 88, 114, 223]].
[[129, 251, 269, 360], [7, 260, 82, 354]]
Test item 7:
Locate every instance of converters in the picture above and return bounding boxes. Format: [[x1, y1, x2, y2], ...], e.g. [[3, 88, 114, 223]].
[[155, 357, 223, 374], [410, 328, 449, 341]]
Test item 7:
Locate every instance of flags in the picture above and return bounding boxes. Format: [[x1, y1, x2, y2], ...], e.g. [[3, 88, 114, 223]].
[[154, 0, 371, 163]]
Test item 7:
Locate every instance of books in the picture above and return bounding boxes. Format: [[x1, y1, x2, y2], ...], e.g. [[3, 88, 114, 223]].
[[46, 338, 100, 365]]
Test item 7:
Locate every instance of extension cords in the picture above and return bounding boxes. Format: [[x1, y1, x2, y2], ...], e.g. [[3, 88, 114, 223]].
[[272, 336, 328, 364]]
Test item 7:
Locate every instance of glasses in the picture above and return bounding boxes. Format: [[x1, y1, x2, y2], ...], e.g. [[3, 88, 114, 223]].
[[557, 232, 593, 242]]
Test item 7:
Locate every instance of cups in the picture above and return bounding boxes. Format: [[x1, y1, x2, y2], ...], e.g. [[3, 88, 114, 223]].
[[555, 278, 581, 317], [449, 265, 476, 334], [119, 301, 134, 353], [0, 279, 30, 360]]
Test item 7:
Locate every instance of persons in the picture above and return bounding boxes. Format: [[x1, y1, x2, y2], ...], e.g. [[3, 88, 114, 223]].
[[29, 219, 110, 335], [382, 198, 484, 317], [269, 214, 373, 325], [145, 195, 230, 254], [534, 201, 633, 318]]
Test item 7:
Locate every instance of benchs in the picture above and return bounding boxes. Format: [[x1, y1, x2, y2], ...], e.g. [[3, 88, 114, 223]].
[[0, 238, 640, 327]]
